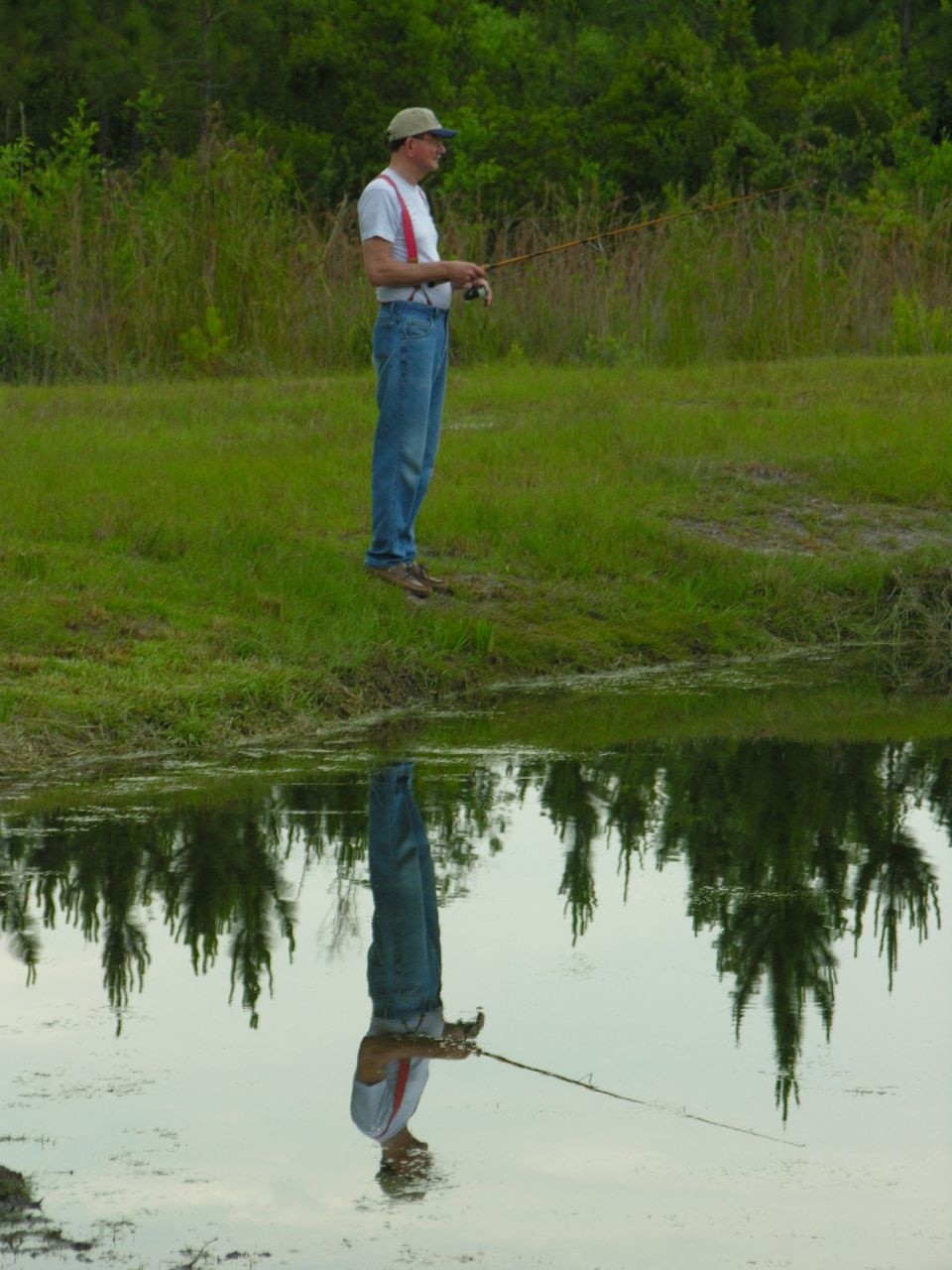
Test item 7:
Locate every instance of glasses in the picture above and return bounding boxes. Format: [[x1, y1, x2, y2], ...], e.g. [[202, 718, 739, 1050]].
[[412, 134, 444, 148]]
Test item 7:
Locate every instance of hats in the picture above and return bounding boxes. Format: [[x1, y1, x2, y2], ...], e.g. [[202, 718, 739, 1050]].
[[386, 108, 457, 143], [374, 1171, 443, 1203]]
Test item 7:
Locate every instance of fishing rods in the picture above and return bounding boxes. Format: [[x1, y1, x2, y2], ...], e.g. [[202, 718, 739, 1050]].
[[461, 174, 828, 272], [451, 1030, 814, 1157]]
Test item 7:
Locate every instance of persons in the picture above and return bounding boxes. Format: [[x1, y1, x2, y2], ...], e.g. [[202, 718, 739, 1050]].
[[357, 107, 493, 599], [350, 760, 483, 1206]]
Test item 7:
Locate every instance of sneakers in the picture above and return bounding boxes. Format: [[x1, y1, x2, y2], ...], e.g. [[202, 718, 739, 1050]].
[[366, 562, 429, 597], [405, 562, 445, 588]]
